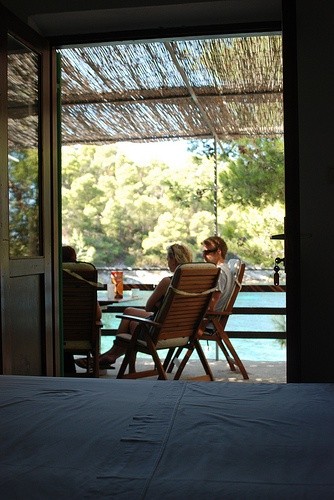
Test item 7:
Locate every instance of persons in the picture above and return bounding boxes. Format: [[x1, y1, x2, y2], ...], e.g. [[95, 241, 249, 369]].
[[62, 246, 101, 351], [201, 236, 236, 335], [74, 244, 191, 370]]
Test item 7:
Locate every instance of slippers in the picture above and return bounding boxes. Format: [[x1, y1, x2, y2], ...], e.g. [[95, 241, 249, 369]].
[[74, 354, 115, 370]]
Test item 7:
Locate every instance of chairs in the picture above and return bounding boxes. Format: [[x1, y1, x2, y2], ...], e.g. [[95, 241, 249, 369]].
[[166, 259, 250, 380], [115, 262, 221, 381], [62, 261, 103, 377]]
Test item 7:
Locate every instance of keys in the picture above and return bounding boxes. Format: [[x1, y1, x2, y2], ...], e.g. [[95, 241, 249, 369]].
[[273, 257, 284, 286]]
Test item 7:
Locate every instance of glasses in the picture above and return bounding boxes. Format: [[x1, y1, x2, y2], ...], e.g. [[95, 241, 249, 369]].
[[202, 249, 218, 254]]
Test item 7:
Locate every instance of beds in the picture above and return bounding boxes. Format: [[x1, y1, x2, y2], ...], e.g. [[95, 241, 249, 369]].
[[0, 374, 334, 500]]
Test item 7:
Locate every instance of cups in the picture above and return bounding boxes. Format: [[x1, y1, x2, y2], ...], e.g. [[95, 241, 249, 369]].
[[132, 289, 139, 298], [107, 284, 115, 300]]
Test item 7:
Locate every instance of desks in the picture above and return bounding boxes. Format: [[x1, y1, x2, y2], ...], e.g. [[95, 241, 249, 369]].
[[97, 298, 147, 376]]
